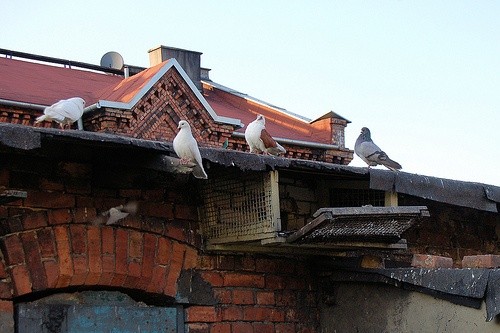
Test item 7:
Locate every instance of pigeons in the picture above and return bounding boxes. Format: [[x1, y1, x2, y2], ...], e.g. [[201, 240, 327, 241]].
[[173, 120, 208, 179], [354, 127, 402, 171], [35, 96, 86, 129], [245, 113, 286, 157]]
